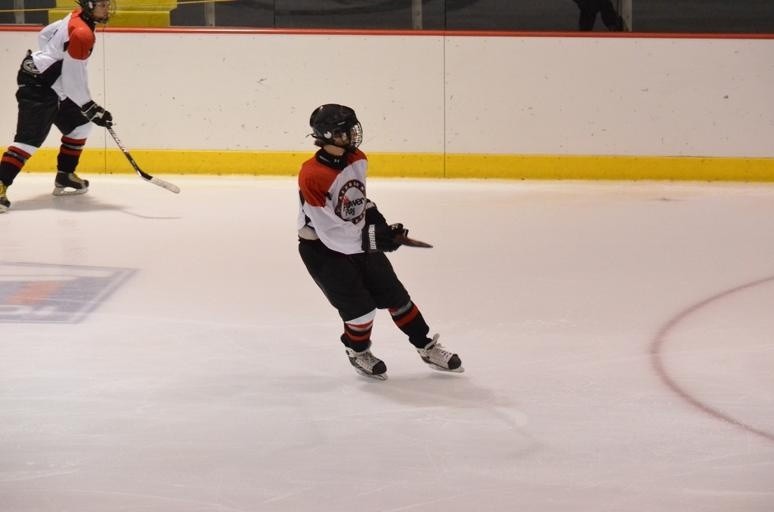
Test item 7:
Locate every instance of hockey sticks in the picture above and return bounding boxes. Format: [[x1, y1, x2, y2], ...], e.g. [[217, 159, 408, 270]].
[[108, 125, 181, 194], [391, 235, 433, 247]]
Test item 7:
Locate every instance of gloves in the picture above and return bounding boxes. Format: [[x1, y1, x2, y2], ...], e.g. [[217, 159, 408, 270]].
[[361, 199, 409, 252], [81, 100, 113, 127]]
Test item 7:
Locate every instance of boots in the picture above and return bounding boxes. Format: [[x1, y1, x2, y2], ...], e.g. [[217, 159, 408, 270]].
[[1, 177, 11, 207], [414, 334, 461, 370], [54, 170, 89, 189], [339, 334, 388, 375]]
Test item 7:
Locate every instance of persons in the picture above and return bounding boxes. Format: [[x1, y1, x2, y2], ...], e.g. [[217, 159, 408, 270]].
[[298, 104, 462, 376], [0, 0, 113, 207]]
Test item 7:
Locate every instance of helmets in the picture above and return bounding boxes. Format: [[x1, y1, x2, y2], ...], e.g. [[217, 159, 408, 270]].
[[310, 104, 358, 145], [74, 0, 100, 15]]
